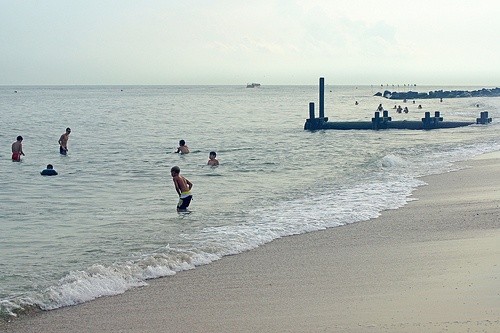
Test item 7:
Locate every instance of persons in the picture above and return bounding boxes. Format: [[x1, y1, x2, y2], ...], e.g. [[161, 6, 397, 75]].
[[176, 140, 189, 153], [40, 164, 58, 176], [403, 107, 409, 113], [208, 151, 219, 165], [58, 128, 71, 154], [171, 166, 193, 211], [355, 101, 359, 104], [377, 103, 384, 111], [418, 104, 422, 109], [393, 105, 397, 109], [396, 105, 403, 113], [12, 136, 25, 162]]
